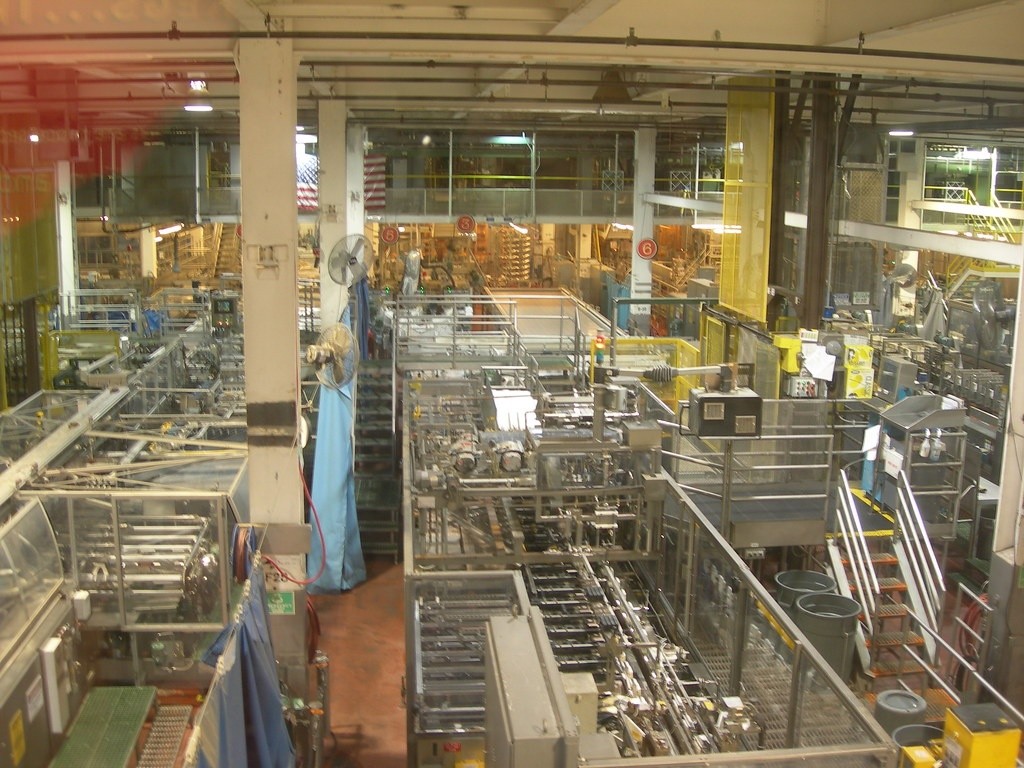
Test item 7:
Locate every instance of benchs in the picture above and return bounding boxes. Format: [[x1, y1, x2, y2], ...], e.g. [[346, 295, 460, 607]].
[[136, 703, 194, 768]]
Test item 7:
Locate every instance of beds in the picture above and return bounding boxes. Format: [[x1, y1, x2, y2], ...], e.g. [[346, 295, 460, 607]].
[[44, 686, 164, 768]]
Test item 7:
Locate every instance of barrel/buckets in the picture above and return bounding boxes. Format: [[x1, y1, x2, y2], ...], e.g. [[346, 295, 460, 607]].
[[795, 592, 862, 685], [774, 569, 837, 623], [892, 724, 944, 752], [873, 689, 927, 738]]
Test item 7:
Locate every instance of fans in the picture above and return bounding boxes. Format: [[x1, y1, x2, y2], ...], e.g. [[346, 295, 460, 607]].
[[326, 233, 375, 287], [892, 264, 919, 289], [300, 321, 361, 391]]
